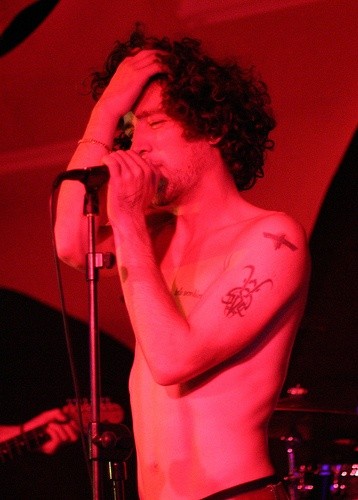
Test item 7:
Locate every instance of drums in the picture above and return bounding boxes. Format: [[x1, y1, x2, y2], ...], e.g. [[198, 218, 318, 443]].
[[281, 461, 358, 500]]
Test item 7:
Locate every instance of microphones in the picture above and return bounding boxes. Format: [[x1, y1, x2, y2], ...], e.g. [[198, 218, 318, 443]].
[[80, 164, 111, 184]]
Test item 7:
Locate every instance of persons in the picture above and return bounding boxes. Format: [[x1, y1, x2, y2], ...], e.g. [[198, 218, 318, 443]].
[[0, 406, 84, 454], [51, 21, 311, 499]]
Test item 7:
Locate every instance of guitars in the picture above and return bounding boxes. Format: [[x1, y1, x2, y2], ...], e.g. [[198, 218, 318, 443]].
[[0, 396, 122, 500]]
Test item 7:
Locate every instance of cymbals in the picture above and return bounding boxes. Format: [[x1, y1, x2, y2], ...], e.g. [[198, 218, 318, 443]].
[[269, 397, 350, 416]]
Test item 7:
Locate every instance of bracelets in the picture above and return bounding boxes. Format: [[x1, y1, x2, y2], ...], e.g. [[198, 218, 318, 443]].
[[19, 424, 24, 434], [78, 137, 112, 151]]
